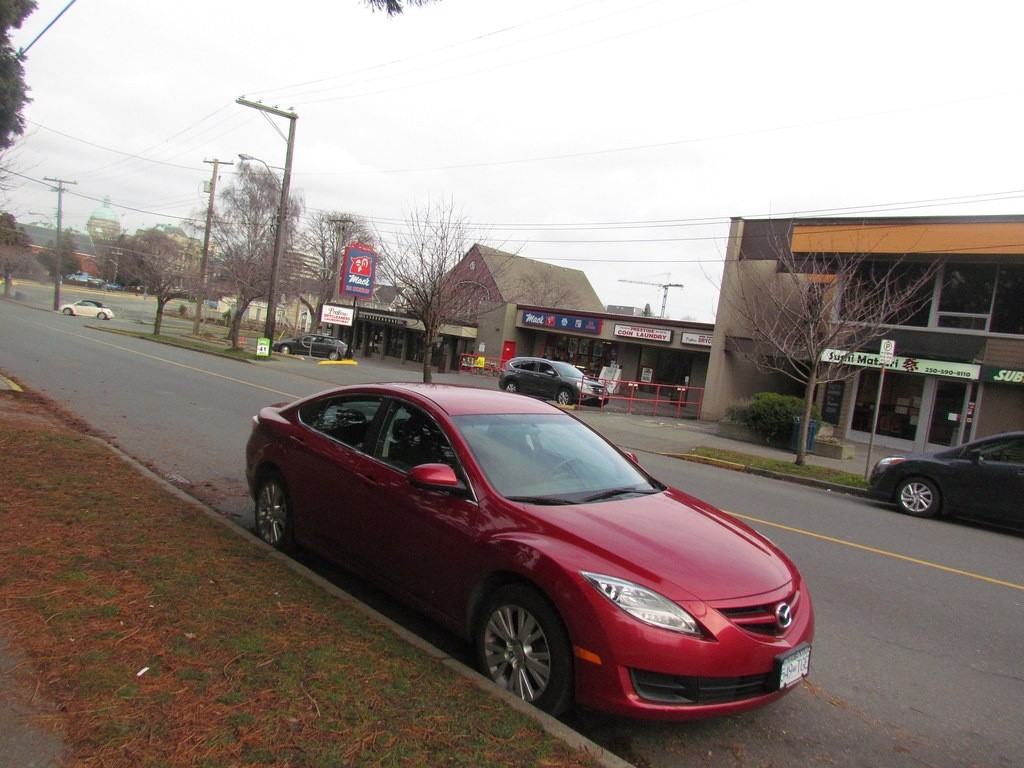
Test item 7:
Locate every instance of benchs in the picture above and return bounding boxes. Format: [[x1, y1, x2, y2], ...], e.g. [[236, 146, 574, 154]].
[[324, 409, 407, 447]]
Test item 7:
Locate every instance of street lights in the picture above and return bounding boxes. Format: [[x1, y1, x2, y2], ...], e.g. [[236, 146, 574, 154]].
[[239, 153, 291, 357], [28, 211, 60, 311]]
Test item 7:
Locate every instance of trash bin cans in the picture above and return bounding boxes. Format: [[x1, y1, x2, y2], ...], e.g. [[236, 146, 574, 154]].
[[787, 416, 818, 454]]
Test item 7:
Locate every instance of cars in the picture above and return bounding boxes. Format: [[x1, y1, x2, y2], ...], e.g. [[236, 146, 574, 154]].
[[60, 300, 114, 321], [273, 334, 353, 360], [868, 431, 1024, 531], [66, 271, 122, 291], [246, 382, 816, 720]]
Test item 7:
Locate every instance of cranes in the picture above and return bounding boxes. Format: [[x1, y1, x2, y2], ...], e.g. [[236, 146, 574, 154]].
[[618, 273, 683, 319]]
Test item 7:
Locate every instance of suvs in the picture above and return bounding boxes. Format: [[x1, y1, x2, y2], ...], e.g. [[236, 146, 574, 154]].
[[498, 357, 609, 406]]
